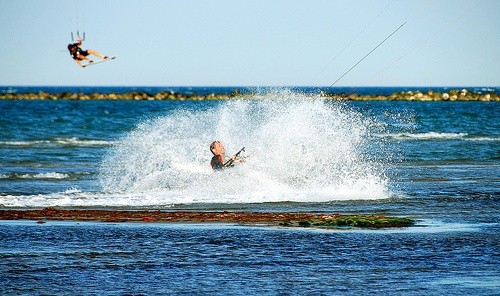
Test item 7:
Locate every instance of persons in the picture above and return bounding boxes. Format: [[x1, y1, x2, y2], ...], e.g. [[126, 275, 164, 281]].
[[210, 140, 246, 171], [68, 39, 109, 67]]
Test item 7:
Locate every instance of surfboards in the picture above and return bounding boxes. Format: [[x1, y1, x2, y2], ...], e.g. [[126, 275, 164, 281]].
[[82, 57, 115, 68]]
